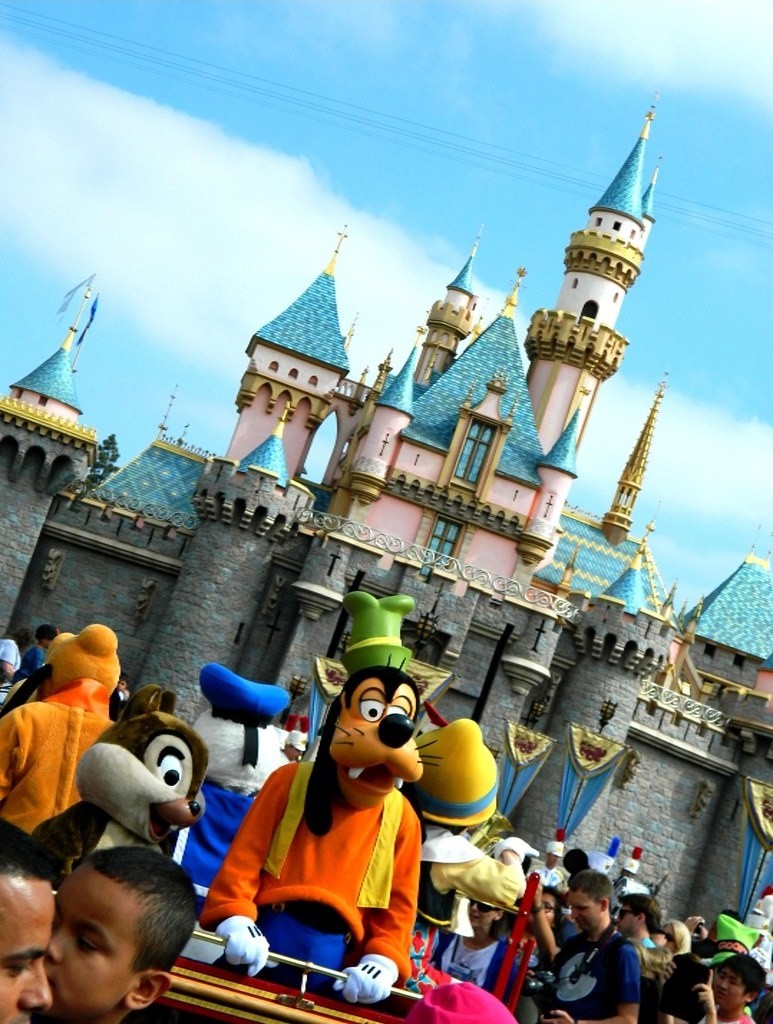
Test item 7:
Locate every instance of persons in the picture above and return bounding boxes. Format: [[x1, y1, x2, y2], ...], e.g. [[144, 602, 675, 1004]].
[[0, 627, 130, 707], [280, 730, 307, 763], [0, 820, 197, 1024], [429, 840, 773, 1024]]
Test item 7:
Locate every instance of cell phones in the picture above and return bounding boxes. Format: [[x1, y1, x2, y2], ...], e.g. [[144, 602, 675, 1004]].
[[672, 955, 710, 984], [692, 919, 705, 937]]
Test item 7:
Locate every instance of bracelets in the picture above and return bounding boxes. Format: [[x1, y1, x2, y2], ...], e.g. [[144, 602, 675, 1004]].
[[574, 1019, 578, 1024]]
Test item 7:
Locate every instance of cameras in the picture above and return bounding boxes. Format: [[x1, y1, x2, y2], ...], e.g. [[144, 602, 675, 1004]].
[[520, 970, 556, 997]]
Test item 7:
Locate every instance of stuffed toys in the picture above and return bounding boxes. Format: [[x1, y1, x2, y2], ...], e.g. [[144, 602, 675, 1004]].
[[200, 591, 421, 1005], [0, 624, 121, 837], [30, 684, 209, 892], [398, 719, 539, 998], [166, 662, 291, 964]]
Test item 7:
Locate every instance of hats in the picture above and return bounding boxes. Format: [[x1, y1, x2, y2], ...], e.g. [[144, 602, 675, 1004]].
[[587, 837, 620, 875], [763, 885, 773, 916], [275, 713, 298, 749], [752, 889, 766, 914], [546, 828, 565, 858], [623, 846, 643, 874], [285, 715, 310, 752]]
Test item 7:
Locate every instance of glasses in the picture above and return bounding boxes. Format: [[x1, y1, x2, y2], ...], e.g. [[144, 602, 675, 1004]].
[[470, 898, 502, 913], [544, 904, 556, 913], [659, 929, 675, 942], [619, 908, 635, 920]]
